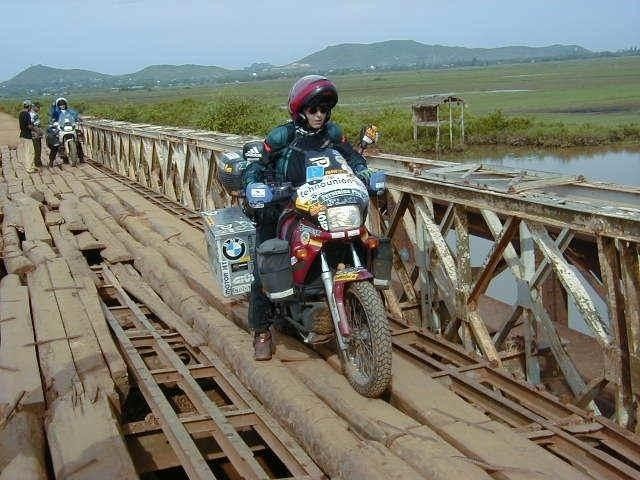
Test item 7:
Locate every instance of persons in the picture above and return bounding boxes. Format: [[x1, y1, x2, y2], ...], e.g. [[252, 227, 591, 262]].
[[48, 97, 86, 166], [18, 99, 40, 172], [29, 100, 44, 167], [242, 73, 377, 361]]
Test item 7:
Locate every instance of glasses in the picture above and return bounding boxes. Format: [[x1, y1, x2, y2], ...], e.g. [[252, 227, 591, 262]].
[[303, 107, 329, 114]]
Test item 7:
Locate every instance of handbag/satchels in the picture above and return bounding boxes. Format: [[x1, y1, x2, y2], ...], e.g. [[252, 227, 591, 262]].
[[255, 236, 301, 306], [46, 128, 61, 151], [365, 226, 393, 290]]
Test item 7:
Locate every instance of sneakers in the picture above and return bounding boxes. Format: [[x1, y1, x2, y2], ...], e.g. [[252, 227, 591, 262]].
[[253, 329, 275, 362], [78, 157, 88, 164], [26, 166, 41, 174], [47, 159, 55, 167]]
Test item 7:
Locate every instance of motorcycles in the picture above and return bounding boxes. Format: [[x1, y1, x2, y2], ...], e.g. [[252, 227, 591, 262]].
[[43, 109, 85, 167], [234, 138, 396, 399]]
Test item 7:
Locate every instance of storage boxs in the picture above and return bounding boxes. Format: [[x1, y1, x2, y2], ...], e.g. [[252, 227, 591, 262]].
[[216, 152, 246, 191], [199, 206, 259, 299]]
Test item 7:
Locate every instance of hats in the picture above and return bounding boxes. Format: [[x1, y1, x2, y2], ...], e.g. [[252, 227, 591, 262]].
[[23, 100, 34, 107]]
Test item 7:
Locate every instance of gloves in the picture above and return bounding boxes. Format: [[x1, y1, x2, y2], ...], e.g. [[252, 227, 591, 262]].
[[245, 182, 272, 210], [355, 167, 377, 185]]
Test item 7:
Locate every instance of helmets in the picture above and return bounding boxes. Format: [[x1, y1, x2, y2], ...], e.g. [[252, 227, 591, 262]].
[[55, 97, 67, 106], [287, 74, 339, 122]]
